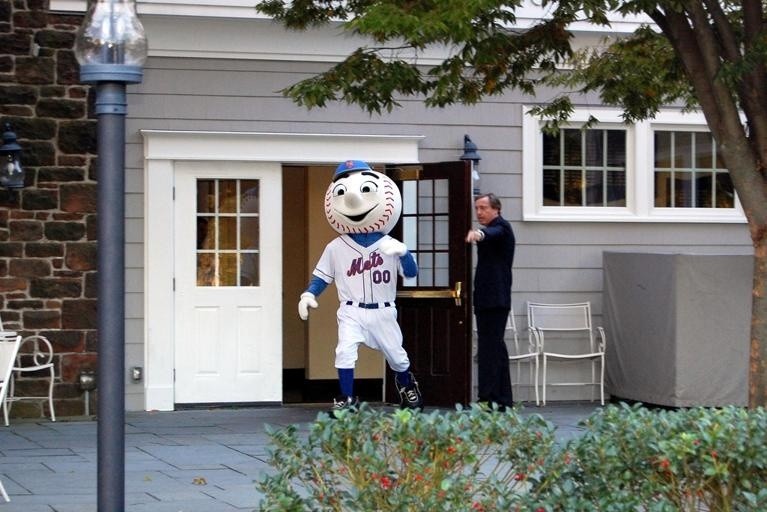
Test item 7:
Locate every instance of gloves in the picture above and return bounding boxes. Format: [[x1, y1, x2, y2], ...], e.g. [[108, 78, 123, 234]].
[[377, 236, 408, 259], [296, 291, 319, 322]]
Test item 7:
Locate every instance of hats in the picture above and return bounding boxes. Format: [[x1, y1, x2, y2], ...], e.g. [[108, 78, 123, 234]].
[[334, 159, 372, 179]]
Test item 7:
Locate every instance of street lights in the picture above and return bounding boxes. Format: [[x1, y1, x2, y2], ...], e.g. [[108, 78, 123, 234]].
[[73, 0, 149, 511]]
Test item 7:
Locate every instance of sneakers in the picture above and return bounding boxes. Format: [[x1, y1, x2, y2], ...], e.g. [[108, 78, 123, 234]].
[[394, 370, 425, 413], [325, 394, 365, 421]]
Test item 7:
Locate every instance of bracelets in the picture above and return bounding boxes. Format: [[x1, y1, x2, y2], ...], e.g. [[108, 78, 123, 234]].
[[477, 229, 484, 242]]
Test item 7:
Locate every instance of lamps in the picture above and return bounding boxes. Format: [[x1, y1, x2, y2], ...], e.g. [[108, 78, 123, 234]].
[[0, 115, 26, 190], [459, 134, 482, 200]]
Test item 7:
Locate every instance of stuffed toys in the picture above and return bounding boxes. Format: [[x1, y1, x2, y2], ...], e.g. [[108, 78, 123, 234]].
[[298, 160, 424, 418]]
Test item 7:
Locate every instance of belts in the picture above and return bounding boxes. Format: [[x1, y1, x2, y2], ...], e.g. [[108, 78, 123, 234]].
[[345, 299, 394, 311]]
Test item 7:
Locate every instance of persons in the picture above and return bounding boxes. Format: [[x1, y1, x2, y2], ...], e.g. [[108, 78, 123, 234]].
[[466, 192, 516, 412]]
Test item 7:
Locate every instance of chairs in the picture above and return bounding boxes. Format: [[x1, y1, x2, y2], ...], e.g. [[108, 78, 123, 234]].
[[471, 300, 606, 407], [0, 318, 56, 503]]
